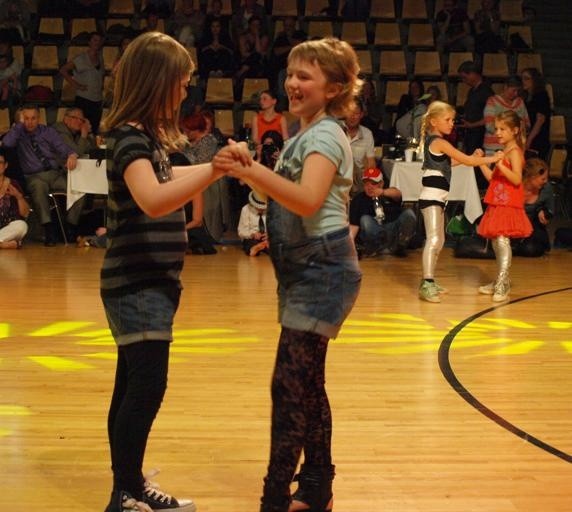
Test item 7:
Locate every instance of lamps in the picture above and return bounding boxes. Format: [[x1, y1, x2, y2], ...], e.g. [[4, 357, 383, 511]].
[[61, 76, 75, 102], [27, 76, 54, 92], [32, 46, 59, 69], [12, 46, 24, 66], [103, 76, 112, 97]]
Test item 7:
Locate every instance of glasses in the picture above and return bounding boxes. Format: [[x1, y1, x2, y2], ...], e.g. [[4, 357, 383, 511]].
[[529, 168, 545, 179]]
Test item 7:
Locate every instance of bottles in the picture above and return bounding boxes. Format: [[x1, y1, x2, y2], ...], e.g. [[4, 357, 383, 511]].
[[394, 129, 401, 146], [244, 123, 250, 141]]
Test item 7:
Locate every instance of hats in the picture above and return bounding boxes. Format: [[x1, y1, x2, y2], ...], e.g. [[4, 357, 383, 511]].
[[360, 167, 383, 183], [249, 190, 267, 210]]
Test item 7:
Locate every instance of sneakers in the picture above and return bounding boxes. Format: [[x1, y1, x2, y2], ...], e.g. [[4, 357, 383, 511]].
[[478, 277, 497, 295], [493, 272, 512, 303], [104, 492, 153, 512], [419, 280, 449, 294], [139, 479, 196, 512], [418, 279, 440, 303]]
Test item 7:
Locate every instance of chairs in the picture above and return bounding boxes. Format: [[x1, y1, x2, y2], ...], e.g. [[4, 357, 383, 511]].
[[241, 0, 265, 8], [282, 110, 298, 128], [423, 81, 449, 103], [242, 111, 256, 128], [39, 18, 65, 34], [467, 0, 482, 20], [499, 0, 524, 22], [374, 23, 401, 46], [379, 51, 407, 76], [242, 78, 269, 103], [402, 0, 428, 18], [508, 26, 532, 49], [106, 19, 130, 30], [482, 54, 510, 76], [272, 0, 298, 17], [490, 83, 505, 94], [205, 78, 234, 103], [407, 24, 434, 47], [369, 0, 395, 18], [550, 115, 567, 144], [456, 82, 472, 105], [108, 0, 135, 14], [385, 81, 409, 104], [1, 134, 67, 246], [354, 50, 373, 74], [174, 0, 199, 13], [517, 53, 543, 78], [140, 19, 164, 33], [546, 144, 568, 179], [70, 17, 96, 40], [37, 108, 47, 126], [0, 108, 10, 133], [206, 0, 232, 15], [447, 52, 473, 77], [274, 21, 284, 41], [102, 46, 121, 70], [305, 0, 329, 16], [341, 22, 368, 46], [434, 0, 444, 17], [67, 47, 89, 62], [545, 84, 554, 109], [414, 51, 442, 76], [308, 22, 333, 39], [214, 110, 234, 136], [56, 107, 67, 122], [185, 47, 197, 70]]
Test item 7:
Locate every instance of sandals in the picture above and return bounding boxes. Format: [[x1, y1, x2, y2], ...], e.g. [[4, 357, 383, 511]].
[[289, 464, 335, 512], [8, 240, 23, 249]]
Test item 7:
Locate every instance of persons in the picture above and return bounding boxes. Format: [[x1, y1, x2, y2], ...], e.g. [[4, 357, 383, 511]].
[[515, 158, 557, 256], [199, 109, 226, 150], [205, 0, 231, 33], [4, 105, 87, 247], [201, 19, 235, 74], [482, 78, 531, 157], [251, 90, 289, 146], [0, 0, 31, 45], [393, 79, 425, 128], [417, 100, 503, 304], [179, 112, 219, 165], [238, 16, 271, 77], [426, 84, 441, 106], [473, 1, 514, 51], [52, 106, 93, 156], [364, 81, 385, 130], [455, 60, 497, 156], [124, 12, 142, 39], [0, 38, 23, 125], [0, 107, 29, 195], [98, 30, 254, 512], [112, 35, 133, 70], [233, 0, 268, 35], [0, 55, 18, 102], [213, 36, 363, 512], [436, 0, 477, 54], [473, 109, 535, 302], [168, 152, 217, 255], [58, 31, 106, 135], [273, 17, 305, 79], [344, 98, 377, 195], [141, 9, 176, 41], [520, 66, 554, 166], [0, 147, 30, 249], [174, 0, 202, 31], [237, 129, 285, 257], [349, 167, 416, 257]]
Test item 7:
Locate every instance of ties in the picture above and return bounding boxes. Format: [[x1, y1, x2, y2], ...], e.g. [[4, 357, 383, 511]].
[[30, 134, 50, 171], [258, 213, 265, 233]]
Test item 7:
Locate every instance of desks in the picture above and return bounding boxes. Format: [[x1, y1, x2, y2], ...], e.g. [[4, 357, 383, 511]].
[[382, 157, 484, 233]]
